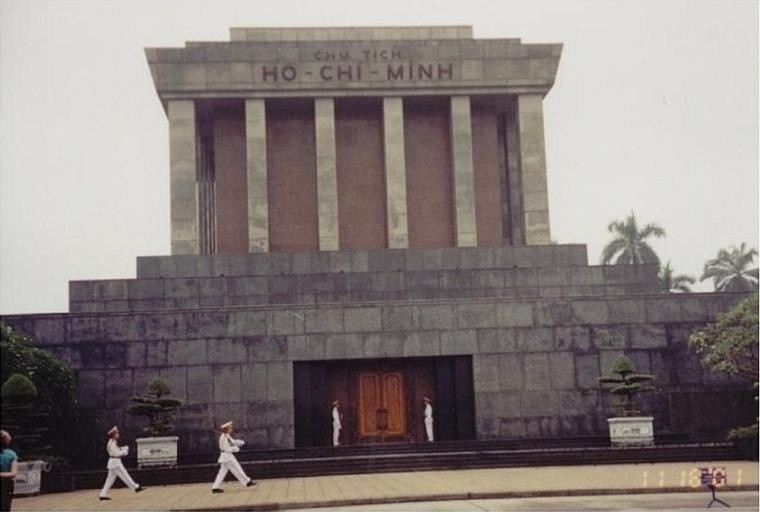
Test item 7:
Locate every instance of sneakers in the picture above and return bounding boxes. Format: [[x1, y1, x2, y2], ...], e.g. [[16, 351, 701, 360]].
[[99, 485, 148, 500], [212, 479, 258, 493]]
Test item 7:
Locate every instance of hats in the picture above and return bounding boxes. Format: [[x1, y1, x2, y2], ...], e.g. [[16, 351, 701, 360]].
[[106, 424, 118, 437], [220, 421, 233, 430]]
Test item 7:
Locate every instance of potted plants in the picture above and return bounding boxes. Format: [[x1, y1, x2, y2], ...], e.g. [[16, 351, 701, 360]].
[[126, 377, 182, 469], [598, 353, 659, 449], [0, 401, 50, 496]]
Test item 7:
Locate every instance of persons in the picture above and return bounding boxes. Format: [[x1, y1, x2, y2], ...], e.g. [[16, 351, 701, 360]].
[[210, 420, 259, 495], [97, 425, 148, 501], [330, 399, 343, 449], [0, 428, 20, 512], [420, 396, 435, 444]]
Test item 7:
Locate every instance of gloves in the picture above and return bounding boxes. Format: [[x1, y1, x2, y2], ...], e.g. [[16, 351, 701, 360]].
[[232, 438, 246, 454], [120, 445, 130, 457]]
